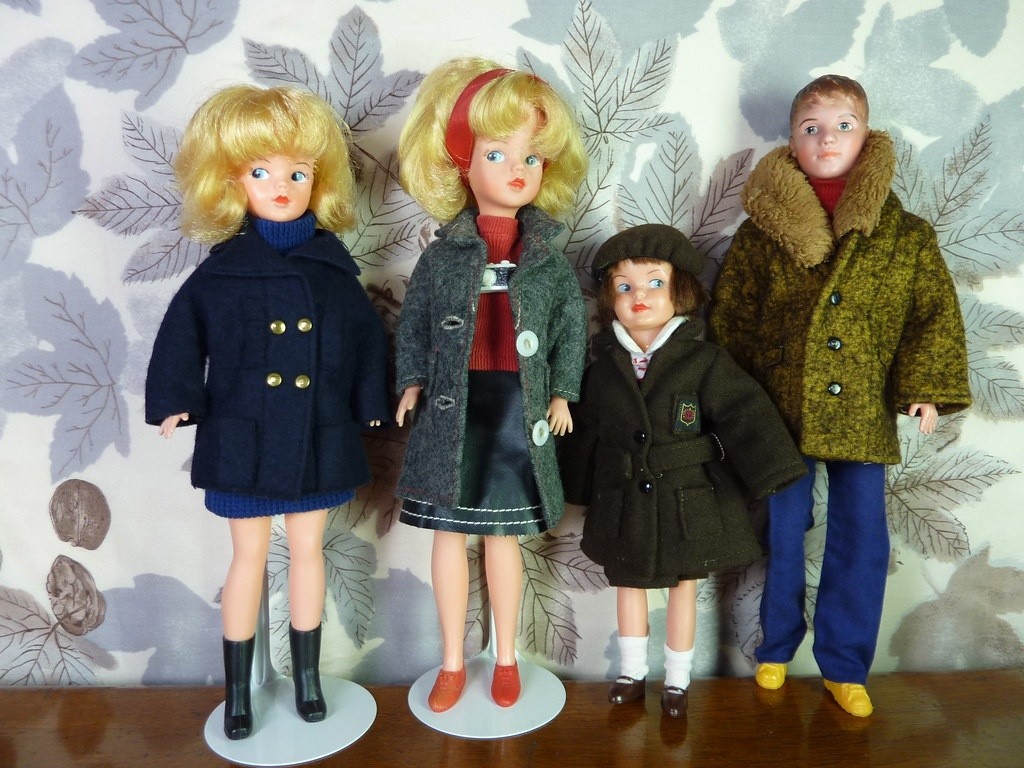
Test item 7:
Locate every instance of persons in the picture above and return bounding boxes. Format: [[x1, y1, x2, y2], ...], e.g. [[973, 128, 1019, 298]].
[[392, 58, 589, 713], [554, 223, 808, 717], [144, 82, 391, 740], [707, 74, 973, 719]]
